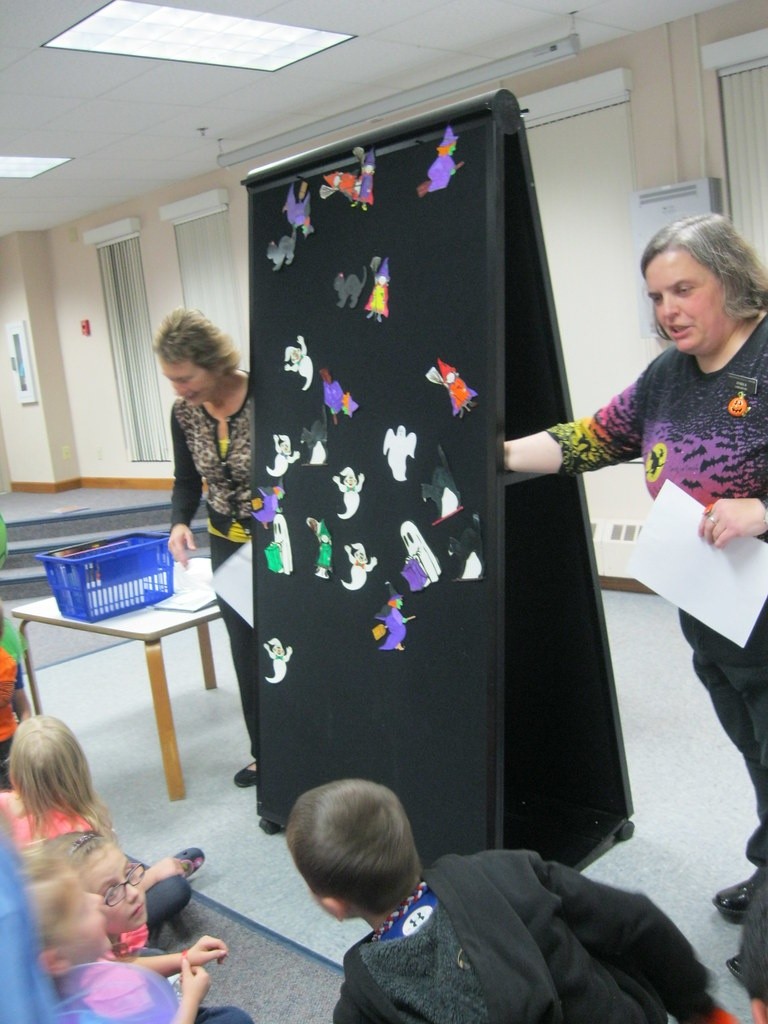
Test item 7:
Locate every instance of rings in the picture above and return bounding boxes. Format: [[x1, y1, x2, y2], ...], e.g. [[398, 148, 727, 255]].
[[709, 516, 717, 524]]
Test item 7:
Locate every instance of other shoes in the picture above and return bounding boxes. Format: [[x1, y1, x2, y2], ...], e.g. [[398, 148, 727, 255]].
[[173, 848, 204, 879], [234, 762, 257, 787]]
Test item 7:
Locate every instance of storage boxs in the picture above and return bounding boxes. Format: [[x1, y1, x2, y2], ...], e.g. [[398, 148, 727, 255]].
[[34, 529, 175, 623]]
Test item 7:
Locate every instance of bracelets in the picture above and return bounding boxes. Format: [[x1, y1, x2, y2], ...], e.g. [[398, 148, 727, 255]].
[[181, 949, 187, 958]]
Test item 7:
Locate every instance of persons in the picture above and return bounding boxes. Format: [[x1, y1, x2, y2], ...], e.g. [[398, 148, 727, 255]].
[[284, 779, 768, 1024], [17, 850, 255, 1024], [153, 307, 257, 786], [0, 517, 205, 957], [503, 212, 768, 982]]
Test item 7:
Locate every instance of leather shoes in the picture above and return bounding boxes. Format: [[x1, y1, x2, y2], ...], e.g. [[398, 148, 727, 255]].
[[726, 955, 743, 980], [712, 881, 754, 915]]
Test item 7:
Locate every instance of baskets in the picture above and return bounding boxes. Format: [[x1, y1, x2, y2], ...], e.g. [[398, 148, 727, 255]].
[[36, 532, 174, 623]]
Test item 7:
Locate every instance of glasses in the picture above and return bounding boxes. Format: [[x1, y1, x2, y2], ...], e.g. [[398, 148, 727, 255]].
[[103, 863, 145, 907]]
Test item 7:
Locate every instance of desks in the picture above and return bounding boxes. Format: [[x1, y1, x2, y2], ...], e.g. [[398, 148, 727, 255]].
[[12, 557, 221, 800]]
